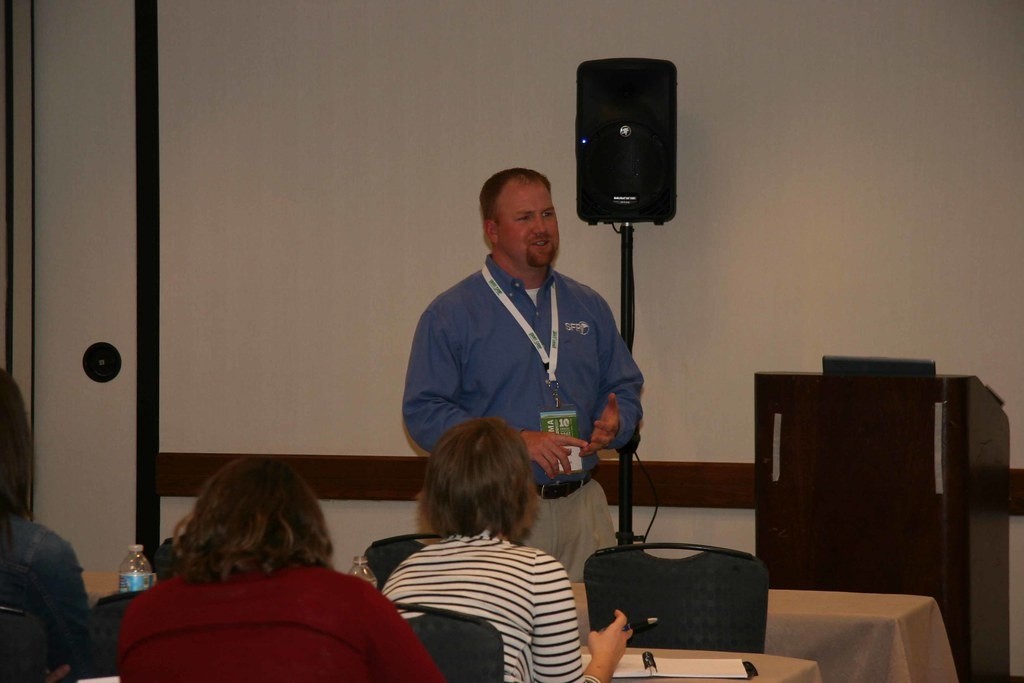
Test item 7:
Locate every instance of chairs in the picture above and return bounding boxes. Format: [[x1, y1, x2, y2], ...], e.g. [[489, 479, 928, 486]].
[[0, 531, 504, 683], [584, 542, 769, 655]]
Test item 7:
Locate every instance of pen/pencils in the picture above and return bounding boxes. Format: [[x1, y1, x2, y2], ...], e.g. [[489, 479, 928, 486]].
[[597, 617, 658, 631]]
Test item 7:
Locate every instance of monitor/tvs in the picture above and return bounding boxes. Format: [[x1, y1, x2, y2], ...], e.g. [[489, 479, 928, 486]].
[[822, 354, 935, 378]]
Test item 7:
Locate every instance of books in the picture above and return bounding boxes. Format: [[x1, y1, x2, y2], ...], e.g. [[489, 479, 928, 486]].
[[580, 653, 759, 680]]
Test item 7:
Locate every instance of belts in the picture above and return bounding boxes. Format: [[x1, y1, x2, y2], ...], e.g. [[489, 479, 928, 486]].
[[534, 474, 591, 500]]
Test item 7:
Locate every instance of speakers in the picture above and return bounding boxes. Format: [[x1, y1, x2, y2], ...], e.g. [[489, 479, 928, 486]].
[[574, 56, 678, 225]]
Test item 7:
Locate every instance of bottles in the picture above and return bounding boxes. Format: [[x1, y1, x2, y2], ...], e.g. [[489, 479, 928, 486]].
[[118, 544, 152, 593], [346, 557, 377, 591]]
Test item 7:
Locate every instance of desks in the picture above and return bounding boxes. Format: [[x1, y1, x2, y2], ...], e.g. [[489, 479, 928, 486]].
[[569, 579, 961, 683]]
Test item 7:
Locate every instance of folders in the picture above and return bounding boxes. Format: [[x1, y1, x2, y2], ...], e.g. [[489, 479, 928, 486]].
[[580, 653, 758, 679]]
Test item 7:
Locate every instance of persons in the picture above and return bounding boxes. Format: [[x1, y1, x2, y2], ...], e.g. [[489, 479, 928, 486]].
[[118, 454, 446, 683], [381, 417, 633, 683], [0, 369, 91, 683], [401, 168, 644, 581]]
